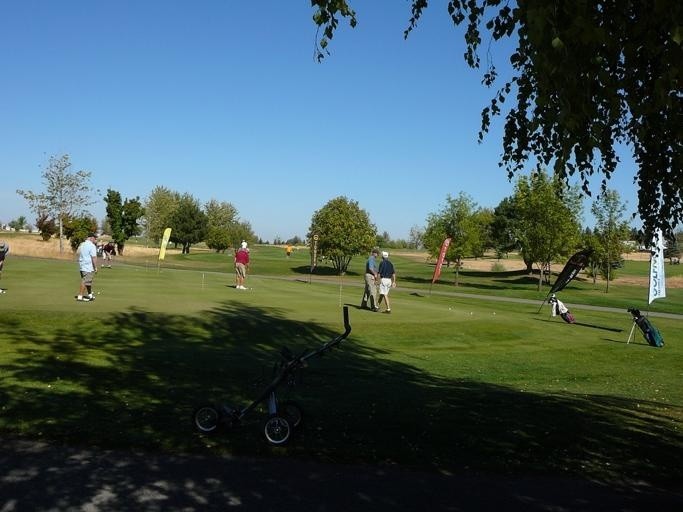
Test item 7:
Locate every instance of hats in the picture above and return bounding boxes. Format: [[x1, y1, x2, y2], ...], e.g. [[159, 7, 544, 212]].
[[241, 242, 247, 248], [382, 251, 389, 259], [88, 232, 97, 238], [372, 249, 381, 255]]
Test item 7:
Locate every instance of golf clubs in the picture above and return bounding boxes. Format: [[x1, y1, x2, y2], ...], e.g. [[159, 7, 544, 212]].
[[247, 271, 252, 290], [628, 307, 642, 319]]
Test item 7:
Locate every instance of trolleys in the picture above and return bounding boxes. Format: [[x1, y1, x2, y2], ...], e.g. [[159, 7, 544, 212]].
[[193, 307, 351, 445]]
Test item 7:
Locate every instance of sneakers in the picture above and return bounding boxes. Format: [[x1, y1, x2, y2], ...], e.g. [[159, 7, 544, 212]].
[[77, 294, 96, 301], [235, 285, 247, 289], [360, 305, 391, 313]]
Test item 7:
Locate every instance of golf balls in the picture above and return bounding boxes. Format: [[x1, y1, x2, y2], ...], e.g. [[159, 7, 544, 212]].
[[494, 312, 495, 314], [0, 290, 6, 293], [449, 308, 451, 310], [92, 291, 100, 295], [470, 312, 473, 315]]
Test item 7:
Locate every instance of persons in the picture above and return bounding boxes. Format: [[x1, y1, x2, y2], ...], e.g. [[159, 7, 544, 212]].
[[94, 240, 120, 257], [234, 240, 250, 290], [360, 248, 381, 312], [285, 243, 292, 259], [373, 251, 396, 313], [0, 241, 9, 295], [100, 240, 114, 268], [75, 233, 97, 302]]
